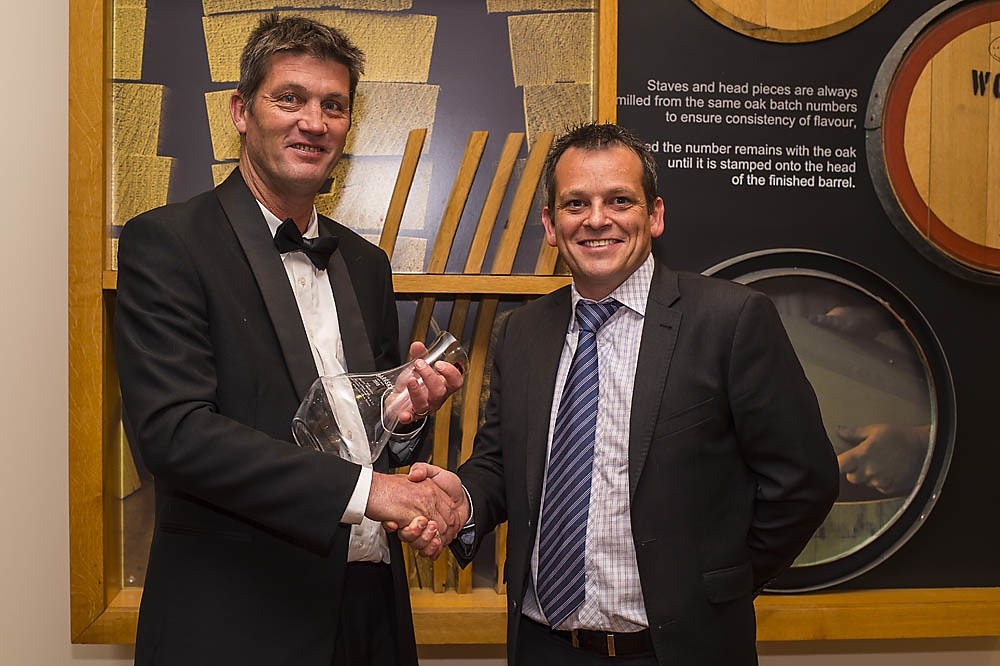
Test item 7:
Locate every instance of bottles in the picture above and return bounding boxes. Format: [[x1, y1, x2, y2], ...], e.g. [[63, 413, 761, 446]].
[[289, 330, 470, 466]]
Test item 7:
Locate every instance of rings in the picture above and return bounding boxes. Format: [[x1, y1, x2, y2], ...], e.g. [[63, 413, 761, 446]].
[[412, 408, 429, 416]]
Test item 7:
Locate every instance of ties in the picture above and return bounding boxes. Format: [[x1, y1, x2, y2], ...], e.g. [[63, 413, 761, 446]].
[[536, 298, 624, 629]]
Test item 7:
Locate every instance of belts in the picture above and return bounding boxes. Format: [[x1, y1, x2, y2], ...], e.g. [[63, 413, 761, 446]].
[[522, 616, 655, 656]]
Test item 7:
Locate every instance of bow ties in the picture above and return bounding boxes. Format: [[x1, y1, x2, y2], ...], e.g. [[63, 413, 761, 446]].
[[273, 218, 342, 271]]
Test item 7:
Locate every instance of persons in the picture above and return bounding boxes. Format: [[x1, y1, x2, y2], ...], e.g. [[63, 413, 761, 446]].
[[383, 120, 840, 666], [113, 7, 461, 666], [813, 302, 932, 497]]
